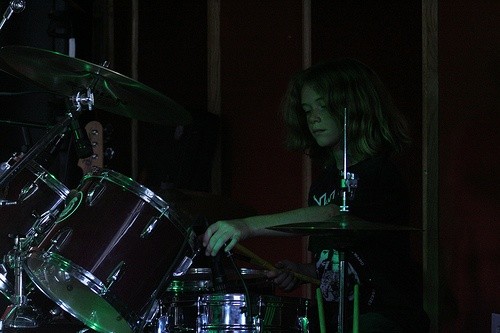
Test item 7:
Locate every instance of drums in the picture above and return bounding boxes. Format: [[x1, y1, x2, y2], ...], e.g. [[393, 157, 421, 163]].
[[0, 147, 72, 304], [153, 265, 272, 333], [19, 163, 206, 333]]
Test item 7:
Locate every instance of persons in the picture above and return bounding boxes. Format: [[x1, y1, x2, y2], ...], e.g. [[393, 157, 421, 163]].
[[202, 48, 434, 333]]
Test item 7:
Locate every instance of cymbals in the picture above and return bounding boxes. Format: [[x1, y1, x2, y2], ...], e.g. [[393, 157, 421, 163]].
[[1, 41, 178, 130], [264, 218, 428, 235]]
